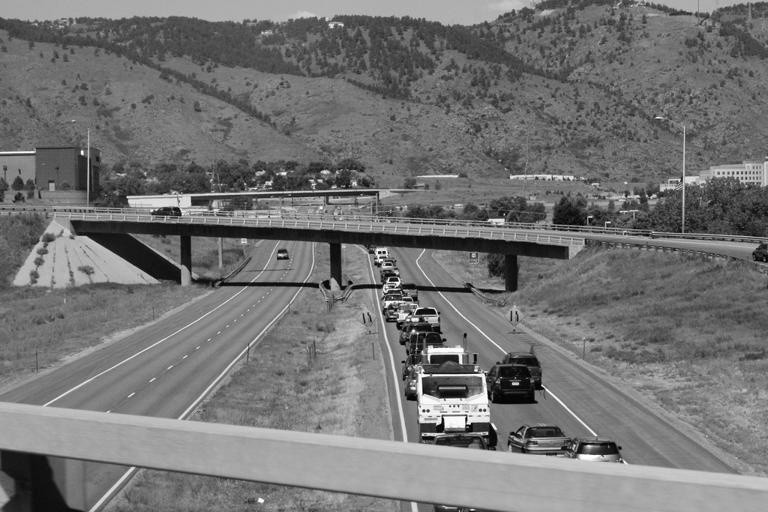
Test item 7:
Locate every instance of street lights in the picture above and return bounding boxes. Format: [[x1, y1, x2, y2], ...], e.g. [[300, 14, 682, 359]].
[[586, 209, 639, 231], [655, 114, 687, 232]]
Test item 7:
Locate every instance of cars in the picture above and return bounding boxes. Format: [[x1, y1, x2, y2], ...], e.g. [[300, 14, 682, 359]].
[[368, 244, 499, 451], [506, 422, 570, 457], [277, 248, 289, 260]]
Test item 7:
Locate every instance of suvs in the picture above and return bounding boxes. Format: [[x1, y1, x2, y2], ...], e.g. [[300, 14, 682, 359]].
[[502, 351, 541, 389], [430, 431, 495, 512], [151, 206, 181, 219], [561, 436, 623, 465], [484, 363, 535, 403], [751, 243, 767, 262]]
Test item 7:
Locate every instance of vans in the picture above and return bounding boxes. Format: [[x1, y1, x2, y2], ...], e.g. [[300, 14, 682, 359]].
[[484, 218, 504, 227]]
